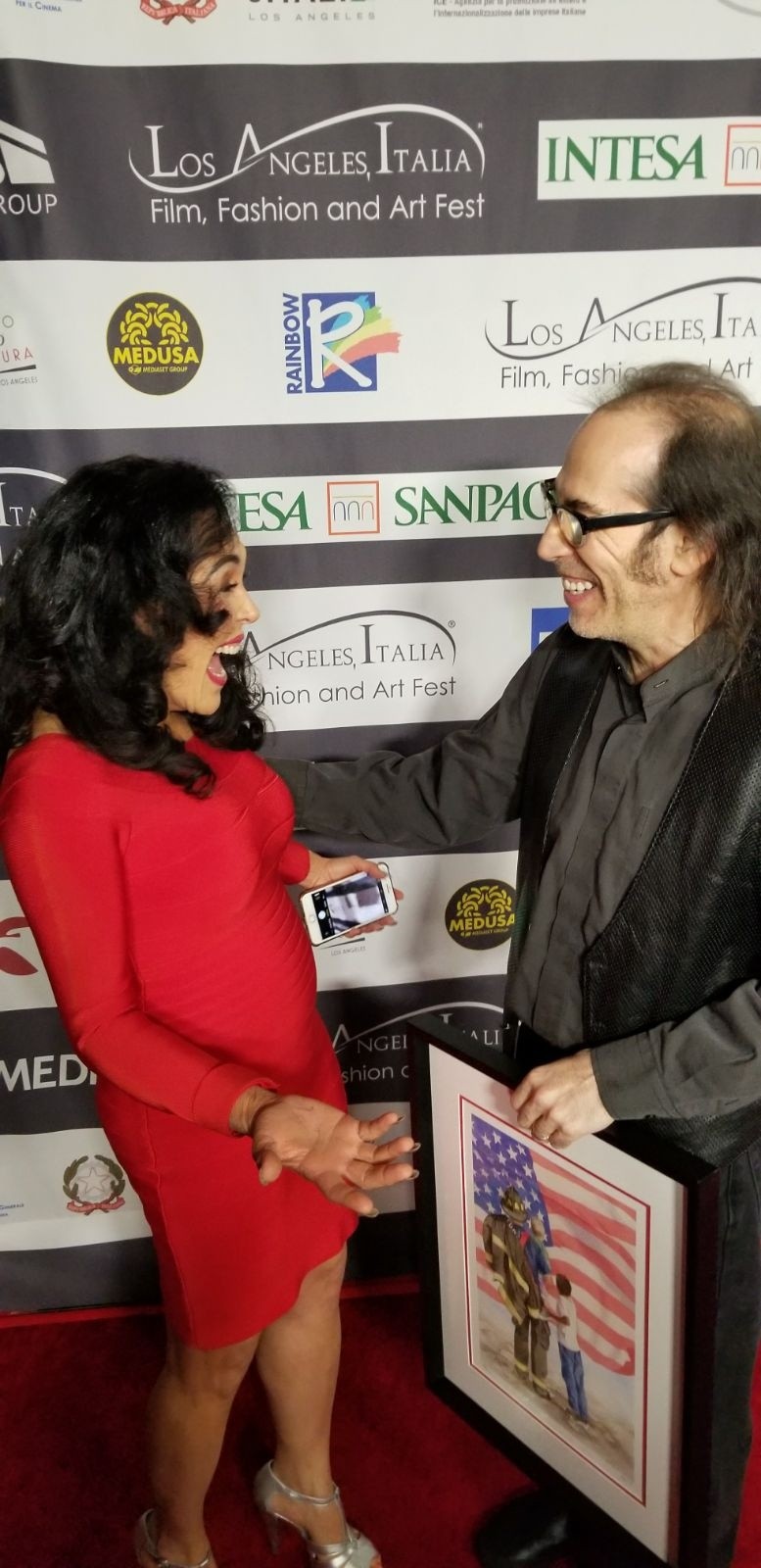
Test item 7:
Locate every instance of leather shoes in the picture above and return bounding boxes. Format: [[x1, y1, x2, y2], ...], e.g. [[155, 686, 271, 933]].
[[471, 1488, 579, 1567]]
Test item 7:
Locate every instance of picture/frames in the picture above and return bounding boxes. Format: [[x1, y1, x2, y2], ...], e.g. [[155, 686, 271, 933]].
[[404, 1006, 721, 1568]]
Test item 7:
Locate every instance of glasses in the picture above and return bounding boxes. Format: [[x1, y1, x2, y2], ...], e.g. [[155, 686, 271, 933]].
[[540, 477, 675, 548]]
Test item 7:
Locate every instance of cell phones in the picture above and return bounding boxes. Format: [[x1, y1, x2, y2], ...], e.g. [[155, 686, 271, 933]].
[[297, 862, 398, 946]]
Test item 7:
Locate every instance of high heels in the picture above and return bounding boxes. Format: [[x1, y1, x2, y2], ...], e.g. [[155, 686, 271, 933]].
[[134, 1508, 217, 1568], [253, 1461, 382, 1568]]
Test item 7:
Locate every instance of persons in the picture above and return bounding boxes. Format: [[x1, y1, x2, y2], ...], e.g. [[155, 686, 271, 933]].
[[0, 454, 419, 1568], [262, 363, 761, 1568], [481, 1187, 593, 1425]]
